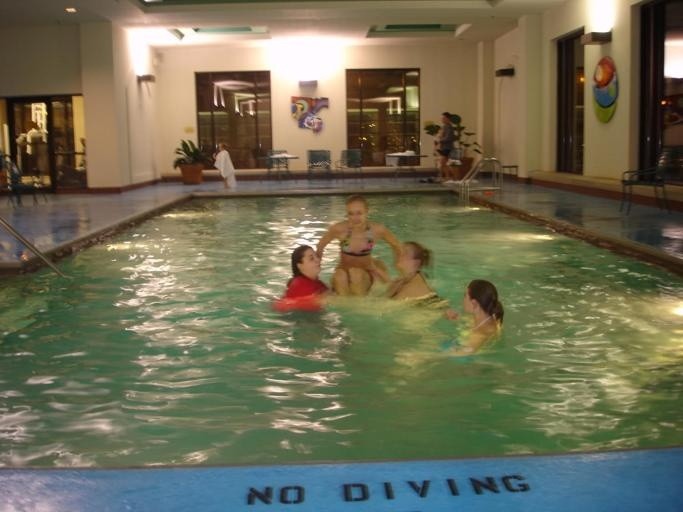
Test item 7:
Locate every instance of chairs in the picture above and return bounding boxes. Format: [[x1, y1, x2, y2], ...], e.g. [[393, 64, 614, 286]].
[[1, 154, 49, 208], [306, 149, 334, 183], [335, 149, 366, 184], [619, 147, 675, 217]]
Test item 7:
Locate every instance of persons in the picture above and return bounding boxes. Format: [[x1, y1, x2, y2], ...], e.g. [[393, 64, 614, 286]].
[[382, 243, 459, 322], [434, 112, 454, 183], [445, 281, 503, 354], [316, 195, 399, 295], [272, 246, 329, 313], [212, 142, 236, 190]]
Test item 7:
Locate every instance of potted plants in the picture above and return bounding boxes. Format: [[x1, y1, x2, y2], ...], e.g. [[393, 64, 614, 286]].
[[424, 113, 483, 181], [173, 139, 211, 185]]
